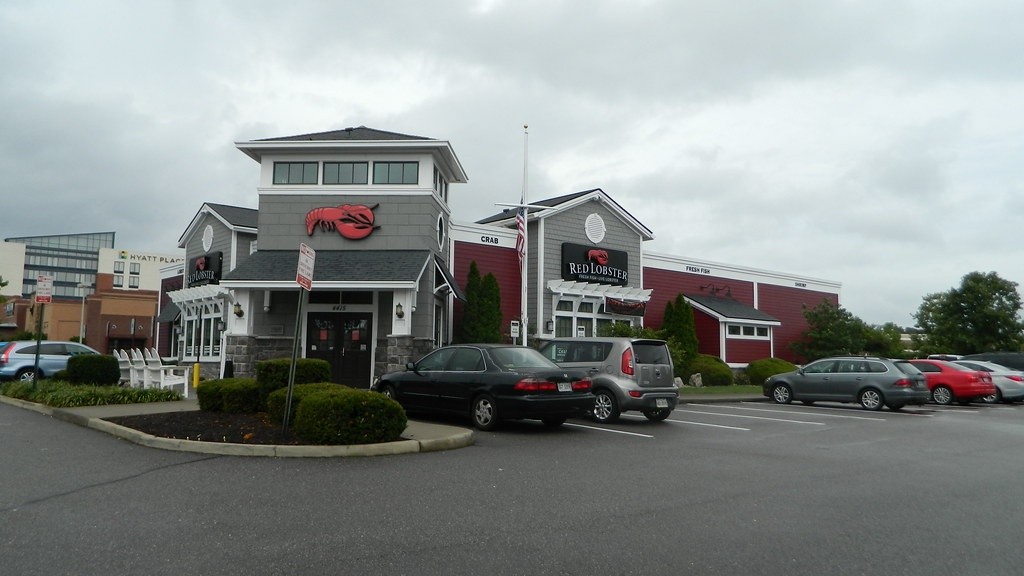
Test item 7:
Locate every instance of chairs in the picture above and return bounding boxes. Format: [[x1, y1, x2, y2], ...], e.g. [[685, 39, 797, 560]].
[[113, 348, 193, 398]]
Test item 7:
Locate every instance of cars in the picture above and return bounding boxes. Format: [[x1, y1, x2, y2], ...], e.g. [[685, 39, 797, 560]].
[[904, 360, 996, 405], [962, 352, 1024, 372], [762, 357, 931, 411], [370, 343, 597, 432], [948, 360, 1024, 404], [0, 340, 102, 384]]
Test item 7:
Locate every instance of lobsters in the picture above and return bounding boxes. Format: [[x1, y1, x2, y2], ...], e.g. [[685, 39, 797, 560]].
[[305, 203, 381, 240], [588, 249, 608, 265], [193, 257, 205, 271]]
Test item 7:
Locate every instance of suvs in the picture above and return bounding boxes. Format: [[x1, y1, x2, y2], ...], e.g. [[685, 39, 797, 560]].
[[513, 337, 680, 424]]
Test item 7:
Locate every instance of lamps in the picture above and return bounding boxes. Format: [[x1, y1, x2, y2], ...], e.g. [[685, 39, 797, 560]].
[[233, 301, 244, 317], [177, 323, 183, 334], [546, 319, 554, 332], [396, 302, 404, 318], [218, 318, 226, 331]]
